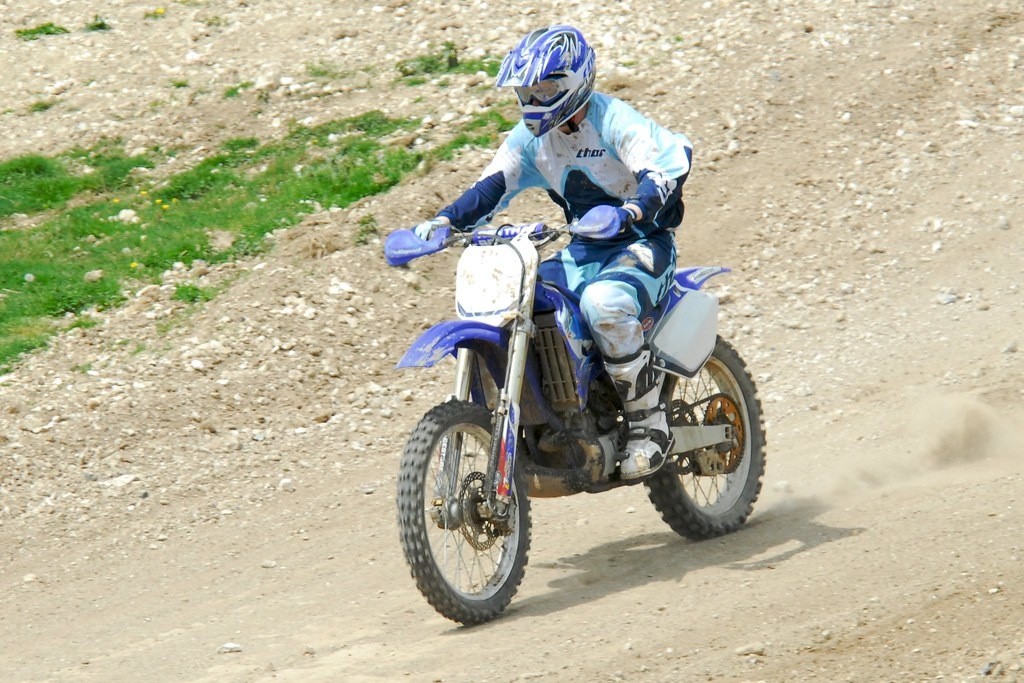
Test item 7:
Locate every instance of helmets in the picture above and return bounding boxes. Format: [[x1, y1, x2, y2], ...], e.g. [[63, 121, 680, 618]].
[[494, 26, 596, 137]]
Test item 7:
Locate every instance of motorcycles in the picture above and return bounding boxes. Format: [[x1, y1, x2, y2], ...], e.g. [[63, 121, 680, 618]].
[[385, 224, 769, 624]]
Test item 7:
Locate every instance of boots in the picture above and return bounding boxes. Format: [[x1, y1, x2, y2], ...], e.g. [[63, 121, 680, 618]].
[[602, 344, 675, 479]]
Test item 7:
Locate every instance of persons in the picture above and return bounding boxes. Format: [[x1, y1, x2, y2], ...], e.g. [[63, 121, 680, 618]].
[[412, 25, 693, 481]]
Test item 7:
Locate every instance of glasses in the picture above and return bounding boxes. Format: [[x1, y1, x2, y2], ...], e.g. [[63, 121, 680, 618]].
[[513, 77, 566, 105]]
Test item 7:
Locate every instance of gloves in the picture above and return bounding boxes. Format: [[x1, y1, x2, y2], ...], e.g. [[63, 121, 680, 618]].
[[613, 206, 638, 235], [411, 218, 445, 243]]
